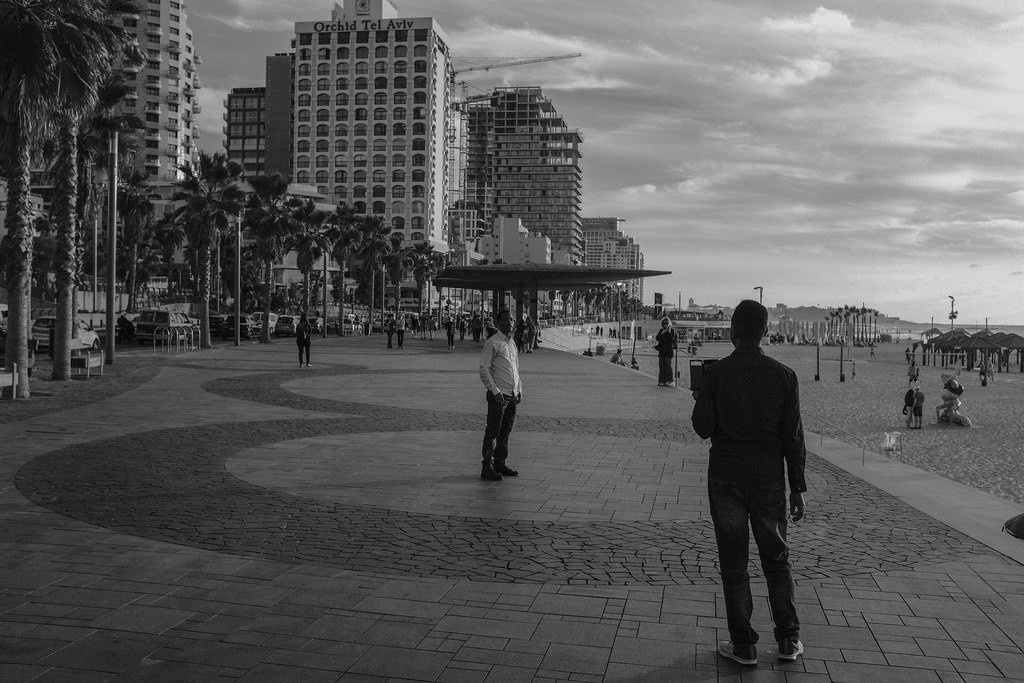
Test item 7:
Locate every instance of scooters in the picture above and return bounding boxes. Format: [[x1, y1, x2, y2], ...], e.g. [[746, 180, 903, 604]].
[[116, 323, 136, 343]]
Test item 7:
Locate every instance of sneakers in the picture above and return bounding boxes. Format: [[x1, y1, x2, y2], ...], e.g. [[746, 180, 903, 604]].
[[780, 639, 804, 660], [720, 639, 758, 664]]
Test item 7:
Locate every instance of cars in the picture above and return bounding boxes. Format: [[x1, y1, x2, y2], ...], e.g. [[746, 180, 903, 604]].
[[308, 317, 330, 333], [336, 314, 388, 335], [188, 312, 228, 335], [31, 315, 100, 351]]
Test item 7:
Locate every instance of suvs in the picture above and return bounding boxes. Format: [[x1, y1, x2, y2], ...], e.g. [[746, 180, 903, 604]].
[[275, 315, 301, 336], [222, 313, 263, 340]]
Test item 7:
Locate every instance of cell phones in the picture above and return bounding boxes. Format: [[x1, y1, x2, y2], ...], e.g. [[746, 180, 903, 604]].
[[703, 359, 718, 371]]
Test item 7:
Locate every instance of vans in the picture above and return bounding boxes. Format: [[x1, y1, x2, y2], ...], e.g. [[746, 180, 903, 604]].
[[404, 312, 419, 325], [136, 310, 195, 344], [253, 312, 279, 333]]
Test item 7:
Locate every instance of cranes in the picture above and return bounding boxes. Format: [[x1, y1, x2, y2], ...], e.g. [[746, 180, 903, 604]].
[[447, 52, 583, 208]]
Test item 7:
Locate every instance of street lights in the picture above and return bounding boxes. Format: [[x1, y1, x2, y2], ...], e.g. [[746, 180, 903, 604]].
[[985, 317, 993, 384], [616, 280, 622, 350], [948, 295, 955, 364], [754, 287, 762, 302]]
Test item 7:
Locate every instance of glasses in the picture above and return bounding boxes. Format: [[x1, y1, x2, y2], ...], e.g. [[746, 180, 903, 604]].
[[663, 322, 669, 326]]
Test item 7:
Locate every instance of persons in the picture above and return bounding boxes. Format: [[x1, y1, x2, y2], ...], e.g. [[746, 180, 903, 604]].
[[850, 363, 856, 380], [478, 310, 523, 480], [553, 313, 638, 371], [905, 389, 914, 429], [692, 299, 808, 665], [295, 313, 314, 367], [868, 342, 876, 359], [687, 331, 807, 354], [912, 388, 924, 429], [656, 317, 679, 386], [904, 347, 1007, 389], [384, 311, 543, 354]]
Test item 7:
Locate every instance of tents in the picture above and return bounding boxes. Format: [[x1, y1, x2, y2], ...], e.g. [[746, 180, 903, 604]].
[[921, 328, 1024, 372]]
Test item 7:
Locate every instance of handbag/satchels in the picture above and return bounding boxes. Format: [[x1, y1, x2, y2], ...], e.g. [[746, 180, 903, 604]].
[[451, 325, 457, 335], [384, 320, 390, 332]]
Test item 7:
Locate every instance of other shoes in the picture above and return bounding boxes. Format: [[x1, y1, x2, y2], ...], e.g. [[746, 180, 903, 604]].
[[481, 466, 502, 481], [308, 364, 312, 366], [494, 465, 519, 478], [657, 381, 665, 386], [300, 365, 304, 367], [526, 350, 529, 353], [667, 382, 676, 386], [528, 351, 532, 353]]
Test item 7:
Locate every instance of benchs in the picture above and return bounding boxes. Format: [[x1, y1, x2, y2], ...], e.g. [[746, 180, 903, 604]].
[[0, 362, 19, 402], [71, 337, 105, 379]]
